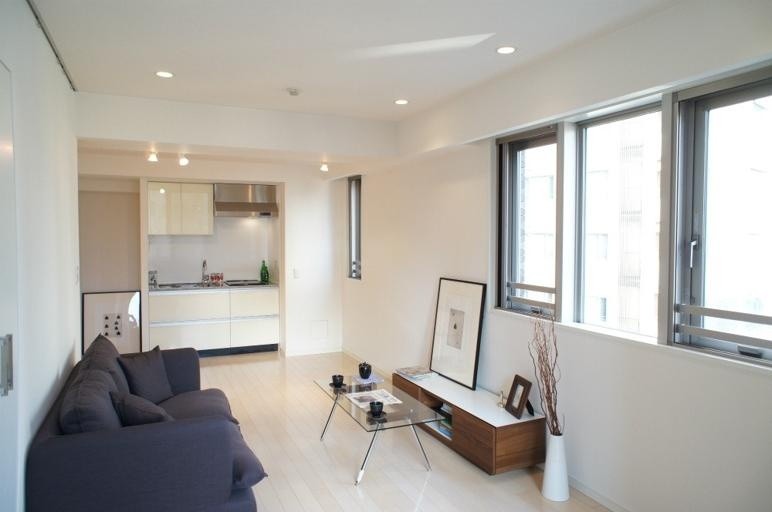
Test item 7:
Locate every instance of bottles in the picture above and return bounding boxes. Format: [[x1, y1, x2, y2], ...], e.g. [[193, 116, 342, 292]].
[[202, 261, 209, 284], [260, 260, 269, 282]]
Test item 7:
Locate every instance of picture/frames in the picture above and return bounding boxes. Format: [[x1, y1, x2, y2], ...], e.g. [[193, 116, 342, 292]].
[[505, 372, 535, 420], [81, 291, 142, 356], [429, 277, 488, 391]]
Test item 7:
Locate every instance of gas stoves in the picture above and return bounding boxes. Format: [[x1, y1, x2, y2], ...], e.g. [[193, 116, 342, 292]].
[[159, 278, 217, 289]]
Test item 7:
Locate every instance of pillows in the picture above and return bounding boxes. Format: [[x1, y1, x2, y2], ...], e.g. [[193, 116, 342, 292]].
[[109, 388, 173, 424], [116, 344, 174, 405]]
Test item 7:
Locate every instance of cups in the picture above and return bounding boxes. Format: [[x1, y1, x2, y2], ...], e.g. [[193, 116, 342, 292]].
[[211, 272, 224, 286], [359, 362, 372, 380], [370, 401, 383, 417], [332, 374, 344, 387]]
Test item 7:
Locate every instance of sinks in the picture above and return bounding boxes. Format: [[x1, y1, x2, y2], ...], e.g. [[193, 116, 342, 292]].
[[193, 282, 223, 288]]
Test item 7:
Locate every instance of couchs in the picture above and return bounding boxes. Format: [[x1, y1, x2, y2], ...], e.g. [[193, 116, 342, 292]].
[[21, 332, 268, 511]]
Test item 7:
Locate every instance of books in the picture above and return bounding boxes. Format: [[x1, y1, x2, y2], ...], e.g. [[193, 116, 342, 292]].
[[440, 403, 453, 438]]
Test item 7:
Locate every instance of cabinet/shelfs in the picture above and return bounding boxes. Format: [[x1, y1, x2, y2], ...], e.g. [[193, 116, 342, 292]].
[[146, 180, 213, 235], [145, 288, 280, 357], [391, 366, 547, 476]]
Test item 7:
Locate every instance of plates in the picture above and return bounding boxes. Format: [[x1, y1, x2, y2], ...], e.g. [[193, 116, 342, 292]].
[[328, 382, 346, 388], [366, 410, 387, 418]]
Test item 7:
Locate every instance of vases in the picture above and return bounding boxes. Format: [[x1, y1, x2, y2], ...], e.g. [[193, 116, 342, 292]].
[[540, 431, 570, 502]]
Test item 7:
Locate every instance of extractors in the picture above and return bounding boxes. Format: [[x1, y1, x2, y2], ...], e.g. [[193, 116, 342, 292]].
[[213, 183, 279, 218]]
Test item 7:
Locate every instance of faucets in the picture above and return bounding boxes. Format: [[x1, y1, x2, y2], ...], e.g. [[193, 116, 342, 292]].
[[201, 260, 208, 284]]
